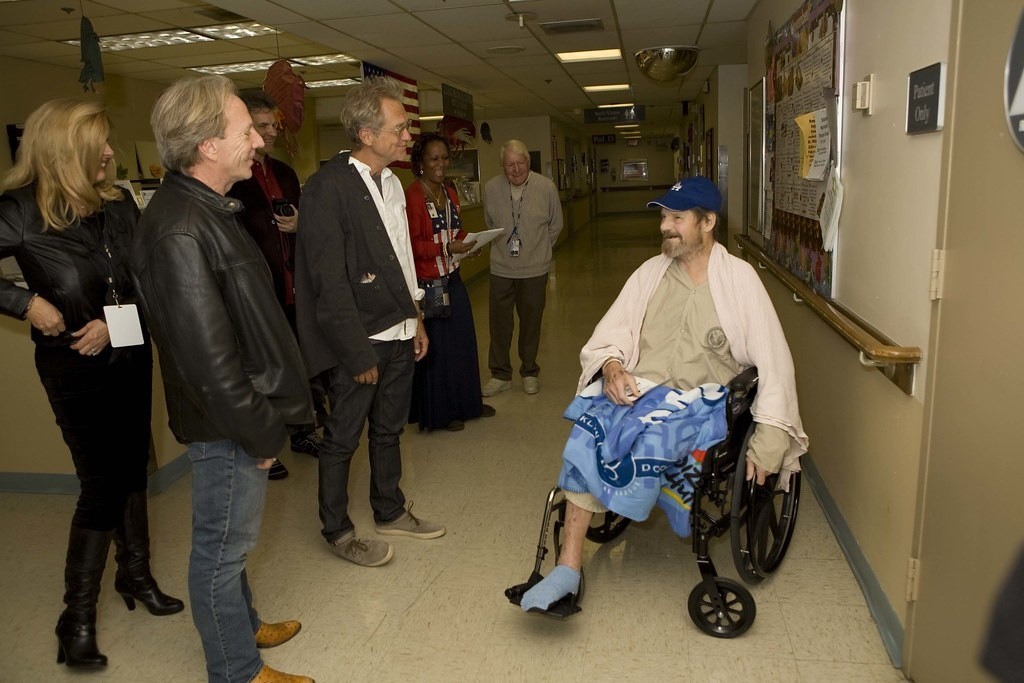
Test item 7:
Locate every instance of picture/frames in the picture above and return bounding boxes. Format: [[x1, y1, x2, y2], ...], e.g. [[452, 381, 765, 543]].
[[558, 159, 564, 191]]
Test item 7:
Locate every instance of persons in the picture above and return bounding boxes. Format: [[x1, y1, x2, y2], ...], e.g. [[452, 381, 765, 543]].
[[482, 140, 563, 396], [226, 89, 322, 479], [406, 132, 496, 432], [521, 176, 811, 611], [138, 77, 317, 683], [295, 81, 446, 567], [0, 97, 185, 668]]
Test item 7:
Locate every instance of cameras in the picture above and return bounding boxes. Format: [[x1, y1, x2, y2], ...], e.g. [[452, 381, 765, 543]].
[[272, 198, 294, 217]]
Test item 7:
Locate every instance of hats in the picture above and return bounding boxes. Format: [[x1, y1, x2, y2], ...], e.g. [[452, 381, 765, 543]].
[[646, 176, 722, 212]]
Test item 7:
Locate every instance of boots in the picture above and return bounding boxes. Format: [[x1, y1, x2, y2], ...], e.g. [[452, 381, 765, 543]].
[[55, 522, 118, 668], [114, 488, 184, 615]]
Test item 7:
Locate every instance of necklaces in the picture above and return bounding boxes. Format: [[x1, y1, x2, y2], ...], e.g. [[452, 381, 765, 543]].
[[419, 178, 442, 206]]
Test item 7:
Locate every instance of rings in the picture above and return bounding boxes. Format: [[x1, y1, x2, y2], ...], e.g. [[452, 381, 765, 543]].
[[44, 331, 49, 336], [91, 348, 96, 354]]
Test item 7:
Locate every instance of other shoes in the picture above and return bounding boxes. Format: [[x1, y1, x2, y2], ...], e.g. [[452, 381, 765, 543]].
[[479, 404, 497, 418], [290, 432, 323, 457], [447, 421, 465, 431], [269, 459, 289, 479]]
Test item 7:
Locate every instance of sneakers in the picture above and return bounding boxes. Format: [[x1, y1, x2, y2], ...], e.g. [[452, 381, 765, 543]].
[[375, 500, 446, 539], [331, 537, 395, 567], [481, 378, 512, 396], [523, 377, 541, 395]]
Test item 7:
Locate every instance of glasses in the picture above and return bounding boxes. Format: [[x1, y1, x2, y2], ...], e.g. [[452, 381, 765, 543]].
[[372, 120, 412, 136]]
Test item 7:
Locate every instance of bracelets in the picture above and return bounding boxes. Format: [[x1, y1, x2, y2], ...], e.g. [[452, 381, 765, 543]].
[[603, 359, 623, 374], [24, 293, 38, 318], [443, 242, 451, 258], [417, 309, 425, 319]]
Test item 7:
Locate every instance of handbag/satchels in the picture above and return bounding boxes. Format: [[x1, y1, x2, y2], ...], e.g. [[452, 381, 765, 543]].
[[418, 279, 453, 318]]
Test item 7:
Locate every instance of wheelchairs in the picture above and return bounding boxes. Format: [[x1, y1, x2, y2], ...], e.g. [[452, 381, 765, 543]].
[[504, 361, 802, 640]]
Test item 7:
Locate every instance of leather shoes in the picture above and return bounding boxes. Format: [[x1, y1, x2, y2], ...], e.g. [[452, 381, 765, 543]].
[[250, 665, 316, 683], [255, 620, 302, 648]]
